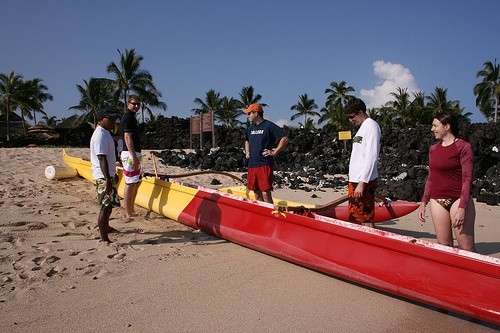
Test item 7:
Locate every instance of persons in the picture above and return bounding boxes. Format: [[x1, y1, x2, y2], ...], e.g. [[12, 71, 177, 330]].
[[344, 98, 381, 228], [243, 104, 287, 203], [90, 107, 118, 243], [117, 95, 142, 218], [419, 114, 476, 253]]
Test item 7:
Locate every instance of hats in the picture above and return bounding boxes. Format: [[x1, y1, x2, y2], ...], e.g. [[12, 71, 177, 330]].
[[242, 104, 262, 113], [99, 106, 122, 118]]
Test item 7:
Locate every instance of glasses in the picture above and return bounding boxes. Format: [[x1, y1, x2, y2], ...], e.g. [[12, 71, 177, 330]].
[[103, 117, 116, 121], [247, 112, 256, 115], [129, 102, 140, 105], [348, 113, 355, 119]]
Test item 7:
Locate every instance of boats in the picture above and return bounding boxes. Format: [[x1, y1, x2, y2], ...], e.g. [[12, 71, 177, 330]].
[[219, 185, 421, 225], [62, 150, 500, 326]]
